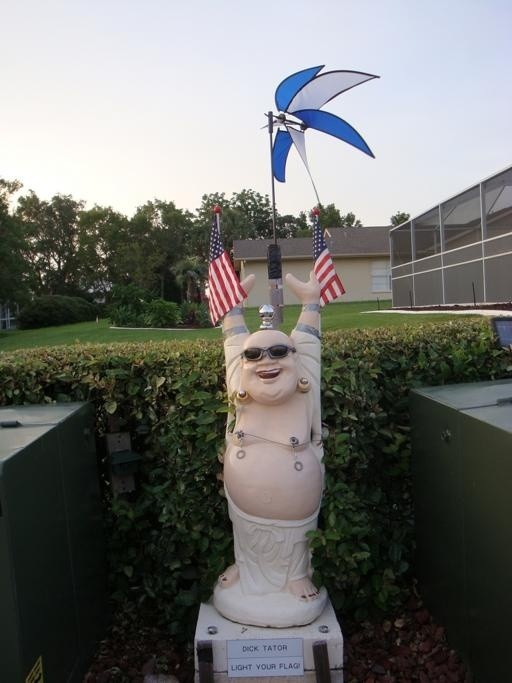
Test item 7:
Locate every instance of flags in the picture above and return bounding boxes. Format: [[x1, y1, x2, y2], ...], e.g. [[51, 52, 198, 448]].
[[209, 213, 247, 327], [311, 216, 345, 309]]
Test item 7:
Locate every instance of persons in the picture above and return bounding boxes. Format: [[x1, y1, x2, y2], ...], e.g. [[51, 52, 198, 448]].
[[221, 270, 323, 600]]
